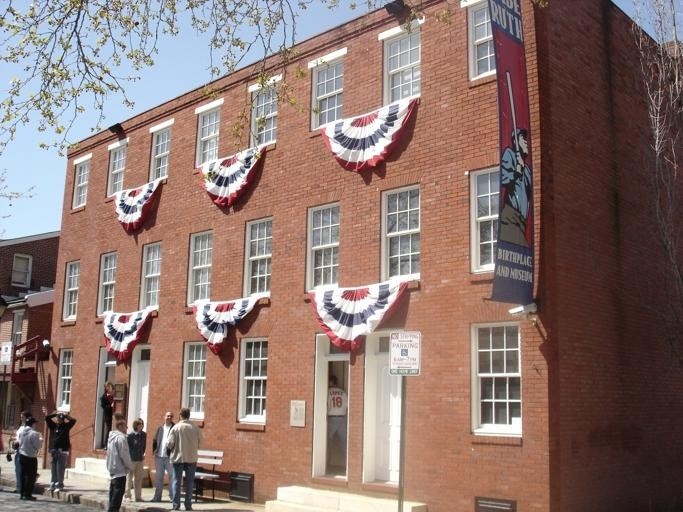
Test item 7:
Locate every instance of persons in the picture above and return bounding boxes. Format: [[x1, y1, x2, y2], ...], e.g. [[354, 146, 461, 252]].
[[327, 375, 348, 465], [9, 412, 42, 501], [44, 413, 76, 493], [499, 128, 533, 248], [106, 417, 147, 512], [150, 408, 201, 510], [100, 382, 113, 451]]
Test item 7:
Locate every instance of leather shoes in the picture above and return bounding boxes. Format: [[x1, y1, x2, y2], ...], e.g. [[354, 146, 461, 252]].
[[123, 497, 193, 512]]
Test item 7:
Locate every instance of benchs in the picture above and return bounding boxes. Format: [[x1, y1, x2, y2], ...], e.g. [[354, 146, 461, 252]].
[[151, 450, 222, 503]]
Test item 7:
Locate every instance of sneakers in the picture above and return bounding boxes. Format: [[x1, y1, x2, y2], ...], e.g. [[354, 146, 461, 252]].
[[13, 484, 64, 501]]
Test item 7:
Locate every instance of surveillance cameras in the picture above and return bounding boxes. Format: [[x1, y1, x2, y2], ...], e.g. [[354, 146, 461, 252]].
[[509, 302, 538, 317], [43, 339, 50, 348]]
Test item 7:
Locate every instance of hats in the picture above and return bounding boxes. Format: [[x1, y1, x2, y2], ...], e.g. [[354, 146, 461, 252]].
[[25, 416, 40, 423], [57, 413, 65, 421]]
